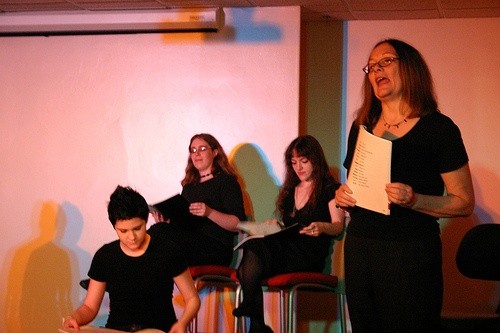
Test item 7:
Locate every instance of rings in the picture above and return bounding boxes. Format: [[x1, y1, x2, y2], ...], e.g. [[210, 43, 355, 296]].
[[311, 230, 314, 234]]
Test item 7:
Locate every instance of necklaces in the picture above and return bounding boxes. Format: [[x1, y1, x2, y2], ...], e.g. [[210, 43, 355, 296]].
[[380, 109, 414, 129], [198, 173, 211, 178], [289, 182, 313, 219]]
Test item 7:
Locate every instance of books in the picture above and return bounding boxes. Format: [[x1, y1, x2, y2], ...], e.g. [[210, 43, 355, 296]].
[[140, 193, 192, 224], [342, 125, 394, 216], [233, 217, 301, 253]]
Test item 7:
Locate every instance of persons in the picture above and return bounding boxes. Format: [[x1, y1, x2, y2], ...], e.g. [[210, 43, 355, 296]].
[[62, 184, 201, 333], [78, 132, 247, 289], [230, 135, 345, 333], [334, 40, 475, 333]]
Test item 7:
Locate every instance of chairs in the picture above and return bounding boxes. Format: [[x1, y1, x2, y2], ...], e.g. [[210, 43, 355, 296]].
[[442, 223, 500, 333], [267, 273, 347, 333], [189, 234, 246, 333]]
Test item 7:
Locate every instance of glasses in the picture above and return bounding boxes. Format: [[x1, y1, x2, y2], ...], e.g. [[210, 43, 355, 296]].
[[190, 145, 212, 153], [362, 56, 399, 74]]
